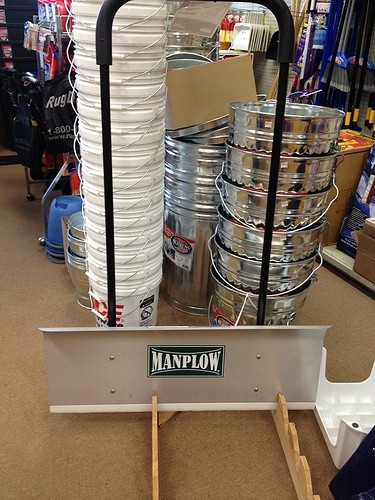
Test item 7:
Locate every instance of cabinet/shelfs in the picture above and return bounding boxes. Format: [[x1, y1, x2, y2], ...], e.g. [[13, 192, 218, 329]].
[[1, 0, 80, 202]]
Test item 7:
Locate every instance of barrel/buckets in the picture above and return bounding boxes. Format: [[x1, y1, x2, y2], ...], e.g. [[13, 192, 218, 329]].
[[44, 195, 82, 265], [164, 95, 268, 317], [207, 100, 346, 327], [64, 1, 168, 329]]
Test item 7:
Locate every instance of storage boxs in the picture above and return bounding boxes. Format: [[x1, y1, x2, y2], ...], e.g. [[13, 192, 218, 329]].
[[323, 151, 369, 246], [348, 229, 375, 284], [165, 53, 259, 131], [362, 217, 375, 239]]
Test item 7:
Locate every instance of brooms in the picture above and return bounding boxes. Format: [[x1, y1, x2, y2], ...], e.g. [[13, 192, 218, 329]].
[[296, 0, 375, 137]]
